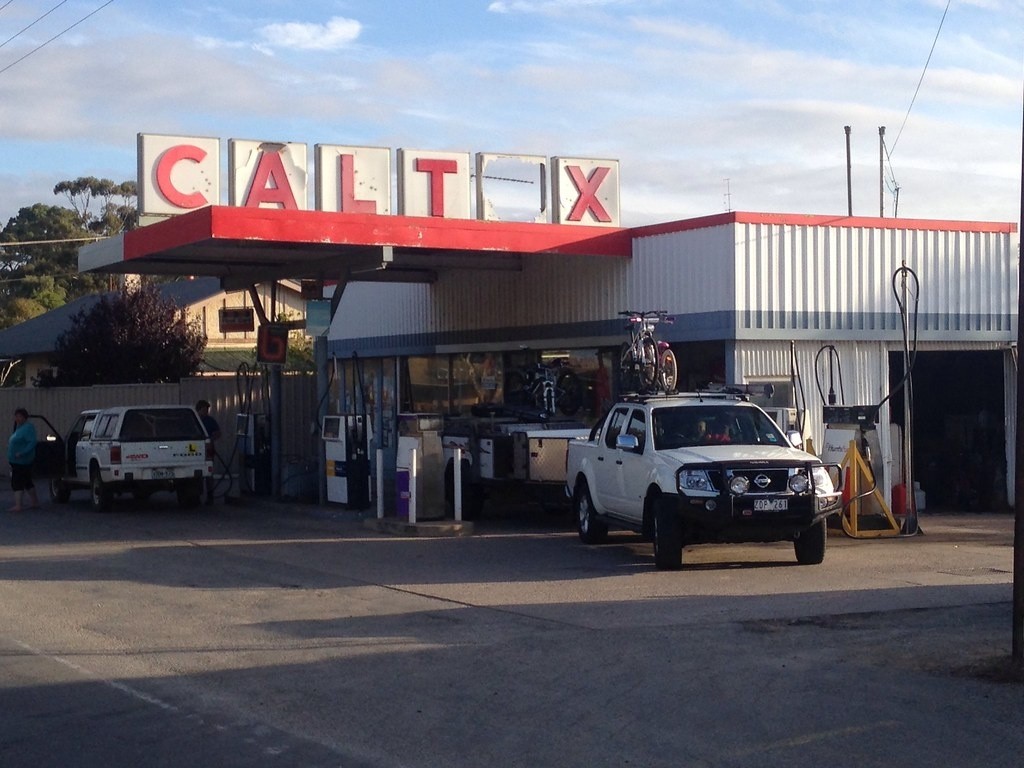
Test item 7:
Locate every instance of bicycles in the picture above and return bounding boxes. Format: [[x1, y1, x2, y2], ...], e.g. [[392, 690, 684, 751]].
[[612, 306, 678, 392]]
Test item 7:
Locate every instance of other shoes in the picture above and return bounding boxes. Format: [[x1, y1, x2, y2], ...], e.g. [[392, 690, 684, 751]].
[[8, 506, 22, 511], [204, 499, 214, 506], [23, 504, 40, 510]]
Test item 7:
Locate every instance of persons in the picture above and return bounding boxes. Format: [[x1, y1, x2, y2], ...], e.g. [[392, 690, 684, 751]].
[[7, 408, 37, 513], [688, 420, 705, 441], [196, 400, 221, 505]]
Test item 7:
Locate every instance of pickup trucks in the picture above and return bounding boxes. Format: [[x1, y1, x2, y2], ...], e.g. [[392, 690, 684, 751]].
[[565, 391, 845, 571]]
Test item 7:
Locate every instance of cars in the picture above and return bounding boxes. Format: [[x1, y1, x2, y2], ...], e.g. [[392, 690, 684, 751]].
[[12, 405, 211, 513]]
